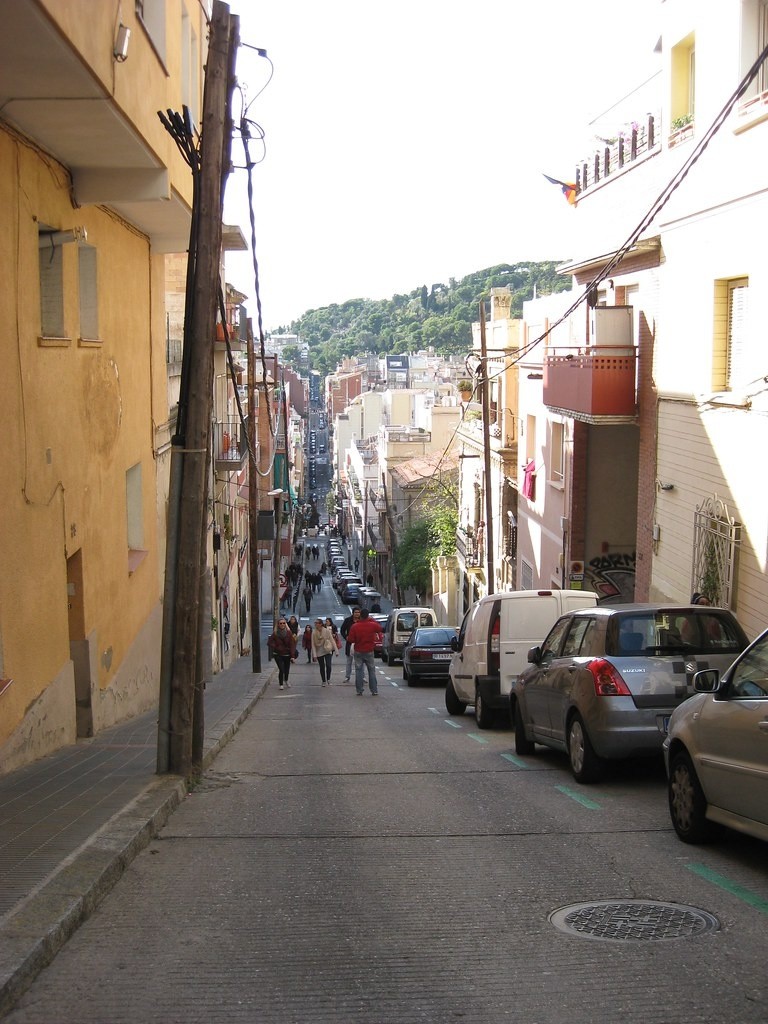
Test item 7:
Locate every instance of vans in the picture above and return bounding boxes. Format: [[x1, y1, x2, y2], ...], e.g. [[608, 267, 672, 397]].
[[445, 589, 599, 729], [381, 607, 438, 666]]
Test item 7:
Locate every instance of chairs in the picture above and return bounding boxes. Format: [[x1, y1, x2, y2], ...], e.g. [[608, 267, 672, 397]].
[[398, 622, 403, 630], [620, 633, 645, 649], [663, 628, 691, 646]]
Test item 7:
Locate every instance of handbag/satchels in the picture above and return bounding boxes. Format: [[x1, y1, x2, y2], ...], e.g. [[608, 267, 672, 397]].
[[289, 649, 299, 660], [324, 640, 333, 654], [334, 633, 342, 649]]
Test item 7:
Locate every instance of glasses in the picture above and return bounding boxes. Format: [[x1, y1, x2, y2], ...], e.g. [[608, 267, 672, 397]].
[[280, 622, 285, 625]]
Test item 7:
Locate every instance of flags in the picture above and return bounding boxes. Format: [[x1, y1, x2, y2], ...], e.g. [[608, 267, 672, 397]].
[[542, 174, 576, 206]]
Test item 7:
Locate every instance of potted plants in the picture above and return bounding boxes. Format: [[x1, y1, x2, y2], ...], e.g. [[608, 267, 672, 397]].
[[457, 380, 473, 402]]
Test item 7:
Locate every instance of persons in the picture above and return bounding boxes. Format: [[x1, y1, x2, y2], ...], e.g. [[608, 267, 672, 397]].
[[322, 562, 326, 575], [294, 544, 320, 561], [287, 616, 338, 663], [346, 608, 382, 696], [340, 608, 368, 683], [354, 557, 359, 572], [286, 563, 302, 587], [305, 570, 324, 595], [303, 586, 313, 612], [681, 593, 723, 646], [367, 573, 373, 587], [287, 586, 292, 608], [269, 617, 296, 690], [311, 620, 339, 687]]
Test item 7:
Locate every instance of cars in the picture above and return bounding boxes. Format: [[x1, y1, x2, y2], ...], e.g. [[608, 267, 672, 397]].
[[319, 413, 324, 429], [320, 445, 325, 453], [509, 603, 751, 784], [310, 375, 318, 402], [403, 628, 455, 687], [310, 429, 316, 489], [663, 628, 768, 845], [326, 538, 389, 658]]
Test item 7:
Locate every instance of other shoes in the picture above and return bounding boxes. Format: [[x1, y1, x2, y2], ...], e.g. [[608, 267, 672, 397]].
[[363, 678, 368, 683], [372, 692, 378, 696], [327, 679, 333, 686], [343, 678, 349, 683], [356, 692, 362, 696], [279, 685, 284, 690], [285, 681, 291, 688], [322, 681, 326, 687]]
